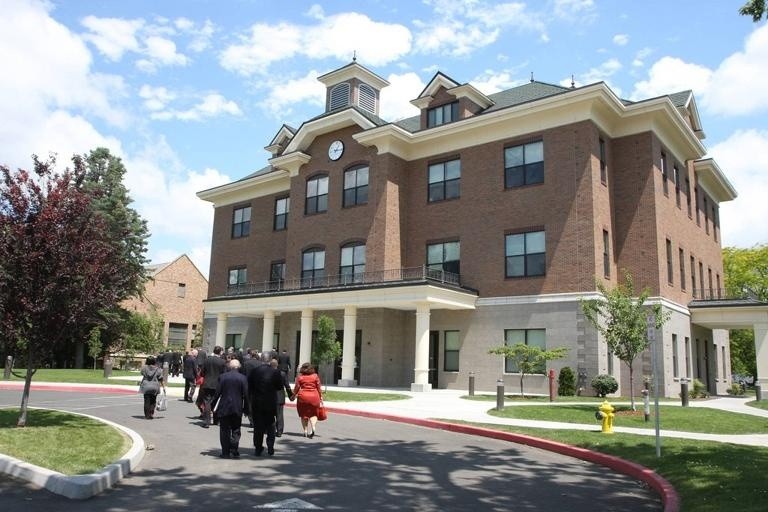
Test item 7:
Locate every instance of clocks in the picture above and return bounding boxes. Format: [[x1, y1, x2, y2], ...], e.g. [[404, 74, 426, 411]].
[[328, 140, 345, 161]]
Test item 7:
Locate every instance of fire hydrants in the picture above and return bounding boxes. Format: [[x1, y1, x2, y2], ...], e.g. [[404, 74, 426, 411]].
[[595, 400, 615, 434]]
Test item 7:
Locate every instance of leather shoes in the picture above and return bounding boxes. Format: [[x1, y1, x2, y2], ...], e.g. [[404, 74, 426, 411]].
[[221, 451, 240, 458]]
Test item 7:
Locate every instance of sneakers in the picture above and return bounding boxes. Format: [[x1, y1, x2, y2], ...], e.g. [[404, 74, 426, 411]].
[[255, 446, 274, 456], [304, 430, 315, 437], [199, 422, 209, 427]]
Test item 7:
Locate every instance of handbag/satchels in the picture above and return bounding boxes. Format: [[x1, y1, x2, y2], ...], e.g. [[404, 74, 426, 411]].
[[157, 398, 167, 411], [196, 377, 204, 384], [318, 407, 327, 421]]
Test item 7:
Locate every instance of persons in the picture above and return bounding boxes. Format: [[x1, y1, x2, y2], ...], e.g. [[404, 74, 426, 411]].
[[289, 363, 323, 438], [141, 356, 165, 419], [157, 345, 291, 459]]
